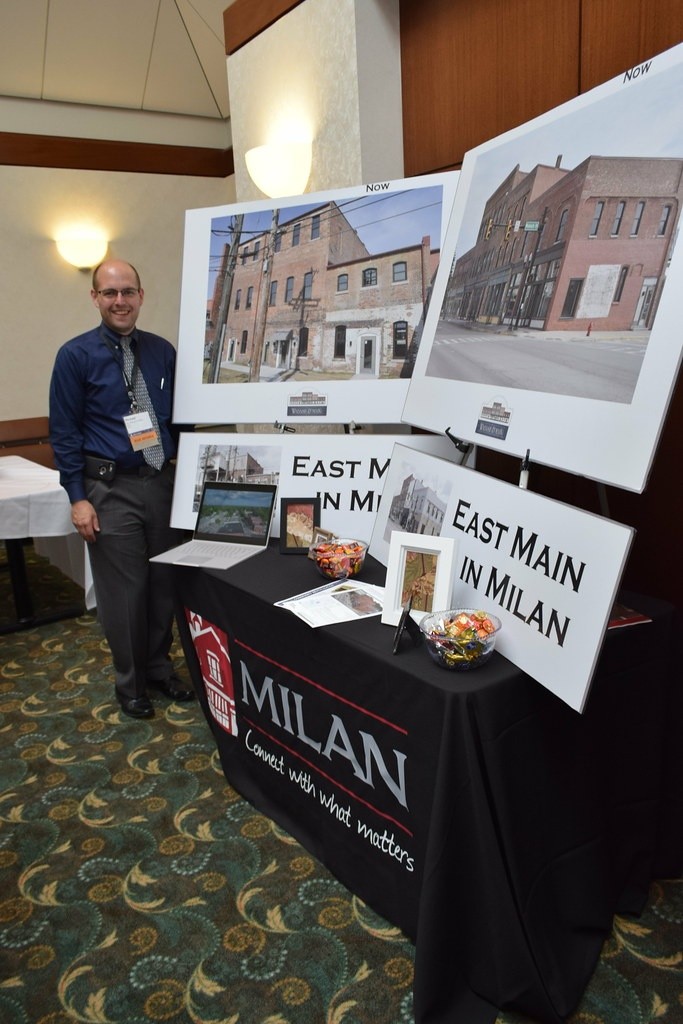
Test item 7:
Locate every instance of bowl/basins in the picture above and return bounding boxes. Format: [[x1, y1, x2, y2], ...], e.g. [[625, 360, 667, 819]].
[[419, 608, 501, 670], [309, 538, 368, 581]]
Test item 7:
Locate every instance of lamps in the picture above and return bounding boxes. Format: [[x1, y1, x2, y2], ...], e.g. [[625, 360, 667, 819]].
[[244, 141, 313, 199], [56, 233, 111, 271]]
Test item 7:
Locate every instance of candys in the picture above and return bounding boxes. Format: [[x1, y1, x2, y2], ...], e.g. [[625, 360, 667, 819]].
[[426, 609, 496, 670], [314, 542, 363, 579]]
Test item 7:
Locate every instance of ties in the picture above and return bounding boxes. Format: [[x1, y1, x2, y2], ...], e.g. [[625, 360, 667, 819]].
[[120, 336, 165, 471]]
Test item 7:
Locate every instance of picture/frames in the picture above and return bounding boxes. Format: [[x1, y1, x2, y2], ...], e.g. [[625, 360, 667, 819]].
[[382, 531, 456, 653], [280, 497, 333, 560]]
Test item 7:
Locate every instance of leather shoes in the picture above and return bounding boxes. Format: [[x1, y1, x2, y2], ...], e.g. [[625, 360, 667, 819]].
[[150, 676, 195, 701], [115, 686, 155, 717]]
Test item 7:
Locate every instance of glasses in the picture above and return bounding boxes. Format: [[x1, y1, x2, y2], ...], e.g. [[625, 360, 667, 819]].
[[97, 288, 140, 298]]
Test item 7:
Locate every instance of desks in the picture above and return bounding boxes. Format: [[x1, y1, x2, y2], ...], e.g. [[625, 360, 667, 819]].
[[160, 539, 683, 1024], [0, 454, 100, 620]]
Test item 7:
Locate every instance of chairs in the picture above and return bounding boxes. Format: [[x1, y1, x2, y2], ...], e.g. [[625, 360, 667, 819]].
[[0, 416, 56, 472]]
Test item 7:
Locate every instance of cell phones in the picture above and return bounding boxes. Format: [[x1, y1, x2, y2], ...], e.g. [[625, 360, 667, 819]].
[[84, 456, 116, 483]]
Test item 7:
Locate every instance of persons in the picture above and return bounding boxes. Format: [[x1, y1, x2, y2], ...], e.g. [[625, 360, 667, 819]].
[[48, 261, 196, 721]]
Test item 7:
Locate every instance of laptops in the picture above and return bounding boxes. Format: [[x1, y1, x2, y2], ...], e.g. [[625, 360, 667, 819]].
[[148, 481, 277, 569]]
